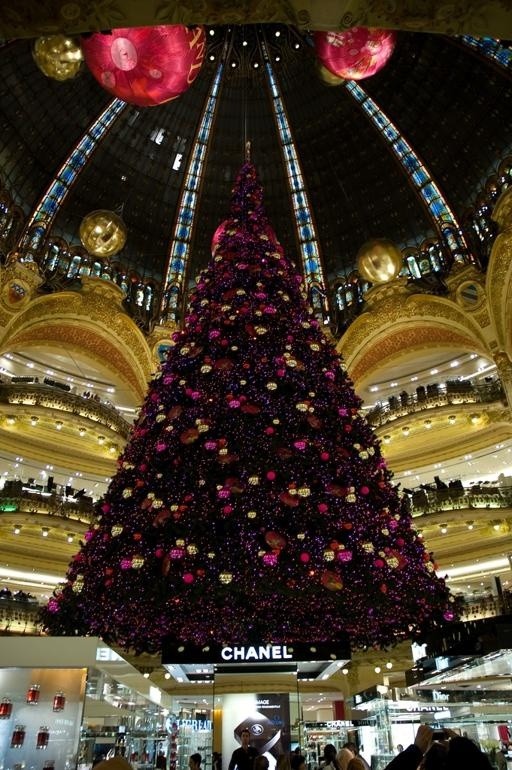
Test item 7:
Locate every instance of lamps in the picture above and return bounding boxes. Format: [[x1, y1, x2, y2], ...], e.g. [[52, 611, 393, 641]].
[[416, 520, 501, 539], [14, 525, 75, 542], [6, 416, 117, 453], [384, 414, 481, 444]]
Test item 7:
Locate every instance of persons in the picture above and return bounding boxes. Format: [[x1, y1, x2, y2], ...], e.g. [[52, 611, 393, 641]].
[[318, 742, 370, 770], [383, 725, 494, 770], [255, 756, 269, 770], [228, 730, 259, 770], [189, 753, 201, 770], [498, 725, 511, 749], [92, 756, 134, 770], [277, 747, 307, 770]]
